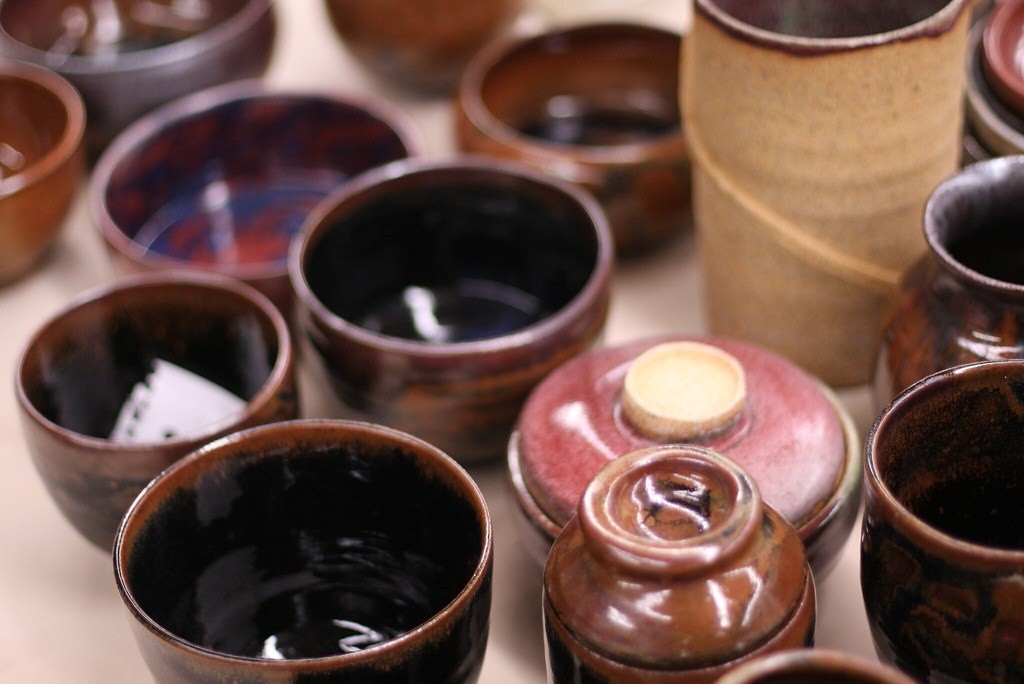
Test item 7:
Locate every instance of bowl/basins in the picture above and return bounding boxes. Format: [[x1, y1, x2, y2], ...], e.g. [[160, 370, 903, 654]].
[[507, 362, 861, 586], [0, 0, 276, 171], [328, 0, 527, 92], [0, 59, 86, 289], [89, 79, 421, 316], [292, 158, 613, 468], [454, 21, 692, 259], [862, 359, 1024, 684], [15, 271, 291, 550], [111, 420, 493, 684], [874, 153, 1024, 411]]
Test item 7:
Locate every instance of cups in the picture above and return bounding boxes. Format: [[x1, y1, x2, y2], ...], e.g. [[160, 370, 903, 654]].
[[542, 447, 814, 684], [680, 0, 967, 389]]
